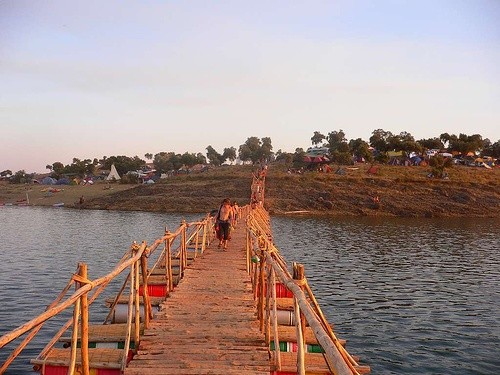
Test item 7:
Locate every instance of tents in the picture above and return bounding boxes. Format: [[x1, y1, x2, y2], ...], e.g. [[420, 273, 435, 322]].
[[56, 178, 70, 185], [30, 179, 40, 185], [41, 176, 56, 185], [106, 164, 121, 181]]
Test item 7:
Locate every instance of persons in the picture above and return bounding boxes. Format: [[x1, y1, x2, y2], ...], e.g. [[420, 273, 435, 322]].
[[216, 199, 233, 253], [259, 162, 269, 171], [73, 176, 95, 185], [285, 163, 322, 175], [218, 198, 242, 242]]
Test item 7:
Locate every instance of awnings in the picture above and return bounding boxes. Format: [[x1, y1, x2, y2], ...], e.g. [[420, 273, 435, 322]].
[[313, 156, 322, 163], [303, 156, 312, 163]]
